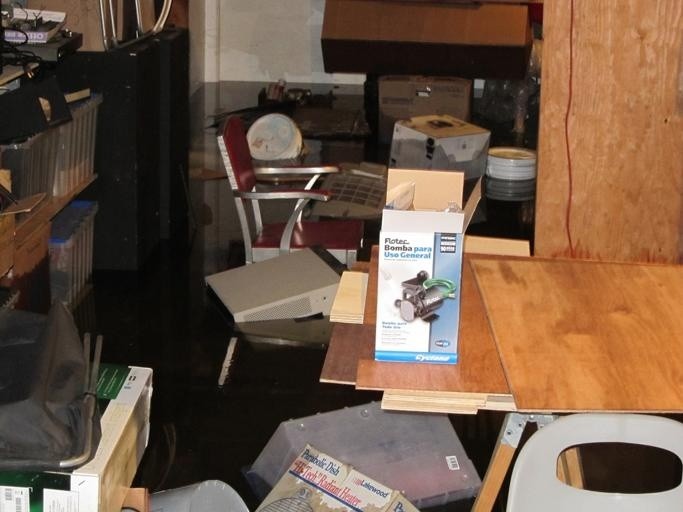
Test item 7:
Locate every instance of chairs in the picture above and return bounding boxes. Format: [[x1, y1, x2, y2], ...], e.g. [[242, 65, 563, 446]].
[[215, 114, 365, 387]]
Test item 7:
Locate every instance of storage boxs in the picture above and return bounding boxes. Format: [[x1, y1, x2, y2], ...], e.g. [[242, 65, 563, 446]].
[[0, 90, 104, 308], [378, 74, 474, 146], [321, 0, 533, 80], [388, 114, 491, 181]]
